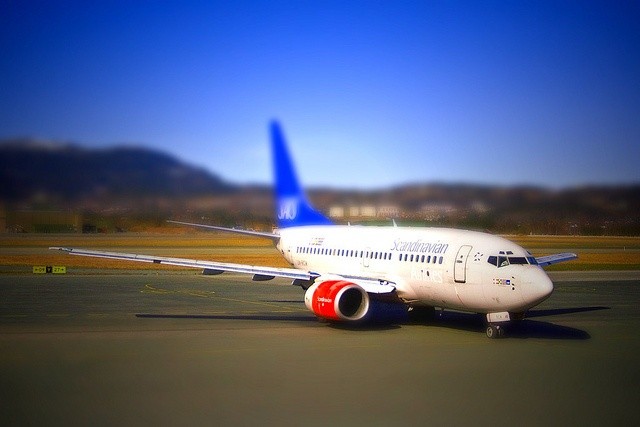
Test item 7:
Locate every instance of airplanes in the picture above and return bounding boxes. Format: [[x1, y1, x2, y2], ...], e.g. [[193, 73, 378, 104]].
[[49, 117, 579, 338]]
